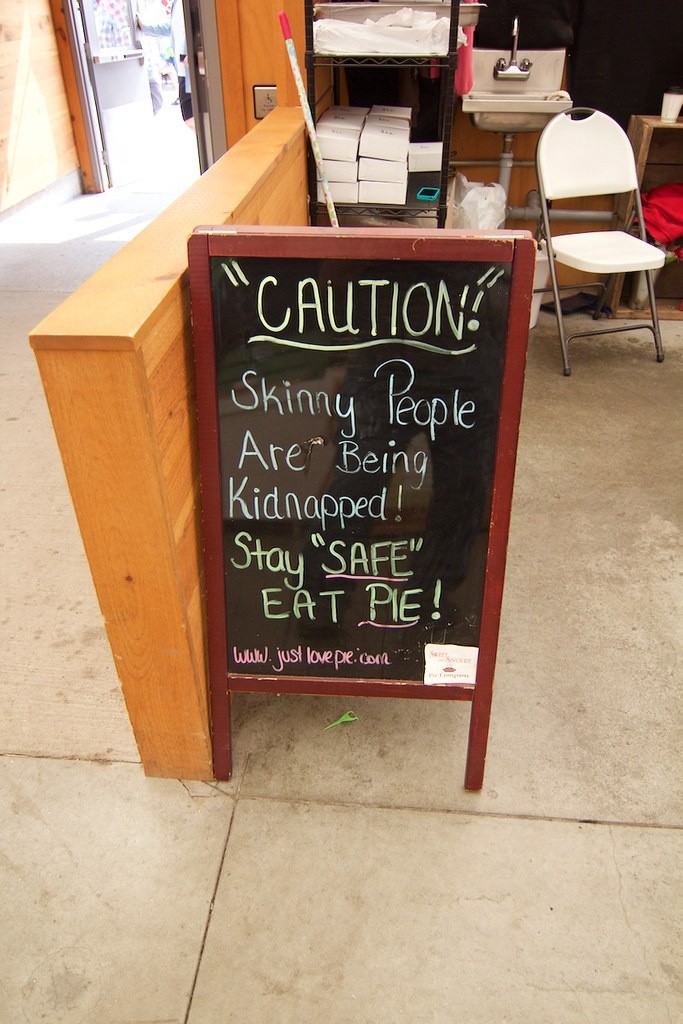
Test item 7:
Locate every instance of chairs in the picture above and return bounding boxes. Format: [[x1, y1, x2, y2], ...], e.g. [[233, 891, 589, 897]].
[[534, 107, 665, 376]]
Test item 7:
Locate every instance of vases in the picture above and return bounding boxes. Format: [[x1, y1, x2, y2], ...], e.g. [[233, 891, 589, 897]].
[[628, 269, 660, 312]]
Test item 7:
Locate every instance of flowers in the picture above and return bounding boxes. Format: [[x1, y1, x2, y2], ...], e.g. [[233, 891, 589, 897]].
[[636, 231, 683, 265]]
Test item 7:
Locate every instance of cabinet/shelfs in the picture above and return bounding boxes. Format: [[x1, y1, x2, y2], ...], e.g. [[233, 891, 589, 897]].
[[305, 0, 461, 228], [602, 115, 683, 321]]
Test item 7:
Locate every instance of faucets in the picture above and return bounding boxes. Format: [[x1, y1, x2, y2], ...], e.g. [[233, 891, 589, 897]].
[[509, 14, 520, 68]]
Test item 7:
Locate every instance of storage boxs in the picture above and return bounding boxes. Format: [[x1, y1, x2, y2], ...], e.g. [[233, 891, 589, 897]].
[[317, 167, 456, 228], [316, 104, 412, 204], [408, 142, 443, 172]]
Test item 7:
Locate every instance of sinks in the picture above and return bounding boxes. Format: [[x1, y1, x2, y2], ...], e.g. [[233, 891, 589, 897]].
[[461, 91, 573, 133]]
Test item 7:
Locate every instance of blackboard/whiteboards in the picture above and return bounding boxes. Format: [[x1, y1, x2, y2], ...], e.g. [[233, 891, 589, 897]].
[[186, 230, 538, 703]]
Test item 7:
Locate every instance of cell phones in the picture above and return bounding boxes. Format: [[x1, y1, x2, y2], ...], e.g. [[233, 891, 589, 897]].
[[417, 187, 440, 201]]
